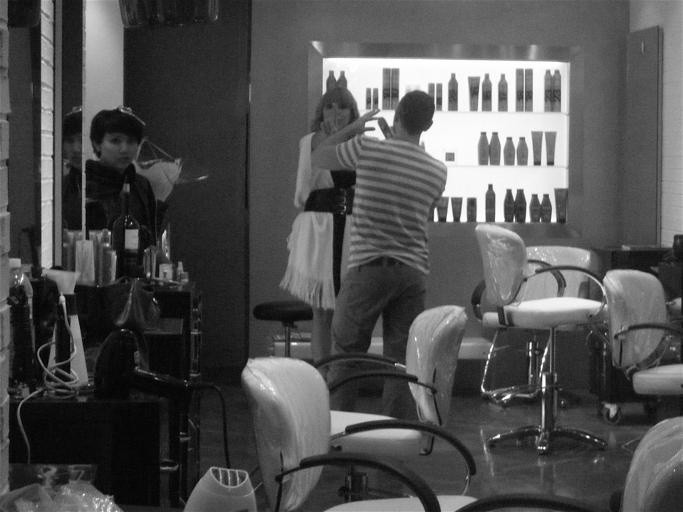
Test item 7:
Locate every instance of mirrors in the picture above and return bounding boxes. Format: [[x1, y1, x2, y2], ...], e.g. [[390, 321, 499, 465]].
[[53, 0, 85, 272]]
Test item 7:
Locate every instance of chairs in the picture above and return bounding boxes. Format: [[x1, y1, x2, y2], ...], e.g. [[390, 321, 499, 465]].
[[602, 263, 678, 421], [457, 417, 682, 510], [315, 305, 466, 503], [242, 354, 477, 511], [469, 246, 600, 407], [475, 226, 610, 457]]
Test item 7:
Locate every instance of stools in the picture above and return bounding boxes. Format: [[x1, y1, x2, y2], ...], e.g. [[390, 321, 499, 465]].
[[254, 300, 312, 362]]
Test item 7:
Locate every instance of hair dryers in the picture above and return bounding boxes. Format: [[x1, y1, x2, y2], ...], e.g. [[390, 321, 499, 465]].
[[183, 466, 259, 512], [93, 329, 190, 400]]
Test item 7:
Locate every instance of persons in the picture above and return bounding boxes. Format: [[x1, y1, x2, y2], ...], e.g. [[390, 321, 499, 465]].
[[86, 106, 157, 248], [310, 90, 447, 419], [280, 85, 360, 363], [62, 105, 82, 226]]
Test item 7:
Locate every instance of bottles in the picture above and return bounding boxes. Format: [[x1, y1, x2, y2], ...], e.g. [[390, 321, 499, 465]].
[[7, 225, 197, 398], [110, 172, 144, 276], [325, 67, 567, 224]]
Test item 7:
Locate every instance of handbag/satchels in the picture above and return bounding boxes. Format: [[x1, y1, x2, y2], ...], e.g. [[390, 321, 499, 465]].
[[97, 276, 161, 331]]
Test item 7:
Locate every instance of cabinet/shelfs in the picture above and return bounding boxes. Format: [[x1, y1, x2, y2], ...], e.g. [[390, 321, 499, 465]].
[[319, 56, 570, 223], [148, 319, 189, 467], [7, 391, 163, 511], [152, 280, 201, 501]]
